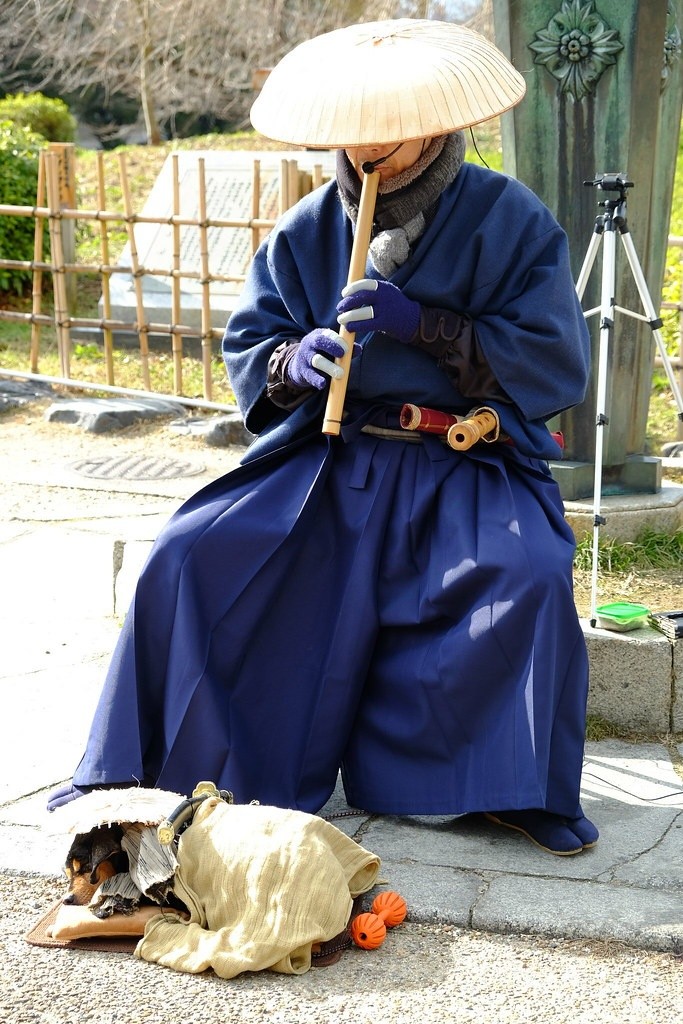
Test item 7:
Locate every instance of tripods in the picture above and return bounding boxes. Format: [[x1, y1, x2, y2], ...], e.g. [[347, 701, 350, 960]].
[[573, 171, 683, 627]]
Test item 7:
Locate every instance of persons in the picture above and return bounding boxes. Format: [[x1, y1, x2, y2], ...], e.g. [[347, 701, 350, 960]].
[[46, 19, 599, 857]]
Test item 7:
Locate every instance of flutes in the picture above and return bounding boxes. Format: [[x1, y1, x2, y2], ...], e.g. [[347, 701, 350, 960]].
[[399, 401, 565, 452], [321, 167, 381, 436], [447, 410, 496, 451]]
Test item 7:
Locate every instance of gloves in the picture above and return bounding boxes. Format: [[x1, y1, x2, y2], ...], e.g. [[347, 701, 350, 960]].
[[336, 280, 424, 343], [286, 328, 347, 389]]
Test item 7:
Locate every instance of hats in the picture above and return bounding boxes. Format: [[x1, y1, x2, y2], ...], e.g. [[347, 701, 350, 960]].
[[250, 19, 528, 149]]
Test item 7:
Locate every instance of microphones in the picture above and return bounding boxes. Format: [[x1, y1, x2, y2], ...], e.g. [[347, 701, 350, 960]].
[[362, 142, 404, 175]]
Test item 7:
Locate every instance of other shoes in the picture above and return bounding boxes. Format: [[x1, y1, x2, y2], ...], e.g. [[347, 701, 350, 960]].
[[46, 784, 92, 812], [490, 809, 600, 856]]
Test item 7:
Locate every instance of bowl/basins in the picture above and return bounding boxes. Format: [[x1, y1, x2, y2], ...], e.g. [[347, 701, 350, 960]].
[[595, 603, 650, 633]]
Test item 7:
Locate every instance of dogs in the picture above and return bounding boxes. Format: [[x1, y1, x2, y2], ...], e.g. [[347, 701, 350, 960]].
[[57, 822, 191, 921]]
